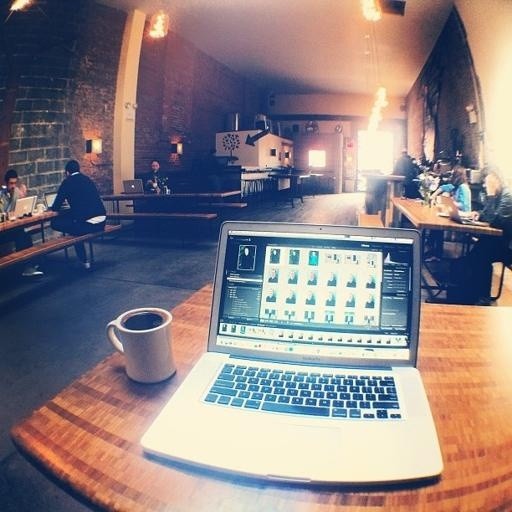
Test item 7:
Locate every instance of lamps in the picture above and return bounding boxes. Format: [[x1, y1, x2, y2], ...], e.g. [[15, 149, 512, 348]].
[[146, 5, 175, 41]]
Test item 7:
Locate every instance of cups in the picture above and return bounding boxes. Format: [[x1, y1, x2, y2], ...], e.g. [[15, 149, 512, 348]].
[[107, 306, 178, 383]]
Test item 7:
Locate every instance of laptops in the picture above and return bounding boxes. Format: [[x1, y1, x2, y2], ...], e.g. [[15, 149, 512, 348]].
[[43, 191, 68, 209], [10, 196, 37, 218], [123, 179, 144, 194], [440, 195, 490, 227], [140, 220, 444, 486]]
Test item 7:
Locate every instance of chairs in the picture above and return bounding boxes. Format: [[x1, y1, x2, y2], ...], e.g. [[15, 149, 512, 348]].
[[241, 173, 307, 209]]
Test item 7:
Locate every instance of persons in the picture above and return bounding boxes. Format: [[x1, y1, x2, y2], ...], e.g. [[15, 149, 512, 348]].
[[262, 265, 380, 311], [51, 160, 107, 272], [0, 170, 44, 278], [394, 150, 511, 306], [143, 160, 172, 195]]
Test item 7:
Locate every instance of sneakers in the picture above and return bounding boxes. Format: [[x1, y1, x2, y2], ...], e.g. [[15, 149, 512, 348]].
[[21, 263, 48, 278], [80, 260, 90, 270]]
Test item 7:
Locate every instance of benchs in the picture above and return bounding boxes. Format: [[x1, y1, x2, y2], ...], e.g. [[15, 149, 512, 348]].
[[0, 223, 123, 279], [126, 201, 249, 209], [103, 213, 218, 223], [25, 223, 53, 238]]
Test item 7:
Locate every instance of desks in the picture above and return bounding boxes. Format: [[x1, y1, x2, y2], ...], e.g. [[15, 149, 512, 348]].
[[104, 189, 242, 202], [1, 205, 74, 250], [9, 280, 510, 512], [361, 171, 509, 306]]
